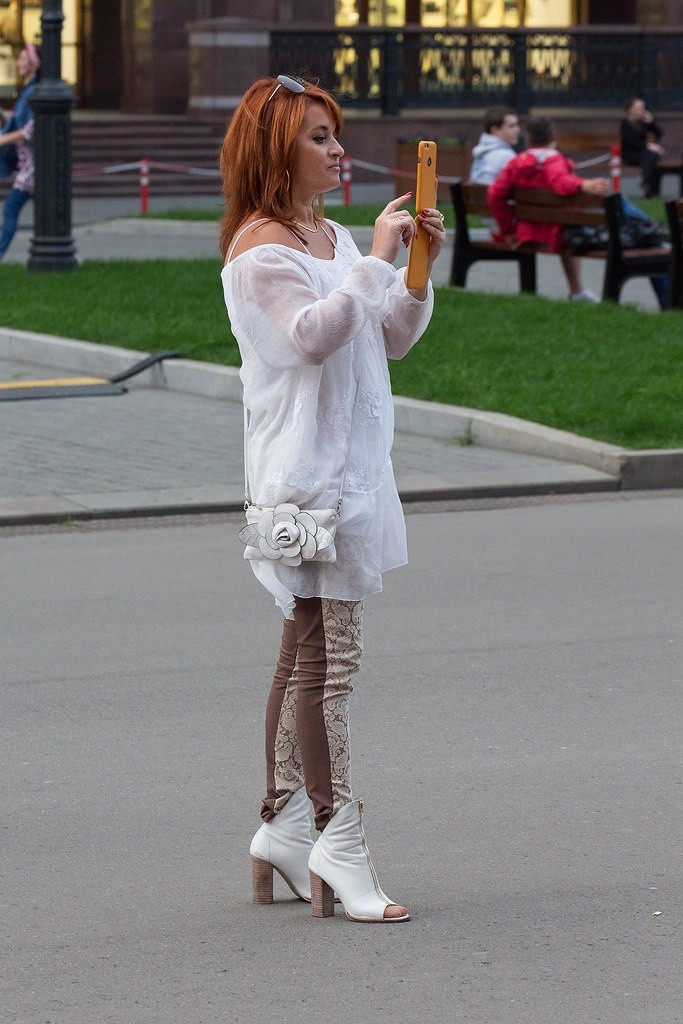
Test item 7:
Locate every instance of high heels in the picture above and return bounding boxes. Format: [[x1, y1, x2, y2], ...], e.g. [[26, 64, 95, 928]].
[[308, 798, 411, 923], [249, 784, 342, 905]]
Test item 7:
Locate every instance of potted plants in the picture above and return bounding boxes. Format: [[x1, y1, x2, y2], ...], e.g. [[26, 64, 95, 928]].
[[394, 129, 473, 201]]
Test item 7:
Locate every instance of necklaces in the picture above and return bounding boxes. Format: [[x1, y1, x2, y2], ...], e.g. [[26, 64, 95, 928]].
[[291, 219, 319, 234]]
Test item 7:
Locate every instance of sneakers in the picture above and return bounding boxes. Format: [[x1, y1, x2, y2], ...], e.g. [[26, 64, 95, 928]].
[[568, 288, 601, 304]]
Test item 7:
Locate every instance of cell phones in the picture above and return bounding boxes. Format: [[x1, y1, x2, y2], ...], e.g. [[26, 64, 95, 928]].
[[416, 140, 437, 214]]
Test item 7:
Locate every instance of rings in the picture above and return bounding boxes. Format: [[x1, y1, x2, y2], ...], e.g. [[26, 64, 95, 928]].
[[397, 216, 405, 220], [440, 213, 444, 222]]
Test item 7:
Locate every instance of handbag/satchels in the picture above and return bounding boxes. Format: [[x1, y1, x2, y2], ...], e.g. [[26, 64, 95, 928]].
[[237, 497, 349, 568]]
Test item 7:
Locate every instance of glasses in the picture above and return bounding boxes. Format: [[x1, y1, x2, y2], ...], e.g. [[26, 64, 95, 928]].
[[268, 73, 311, 103]]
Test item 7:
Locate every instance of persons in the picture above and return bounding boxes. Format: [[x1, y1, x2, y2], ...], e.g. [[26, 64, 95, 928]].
[[620, 96, 665, 199], [219, 76, 446, 925], [469, 106, 601, 302], [0, 44, 42, 260], [485, 113, 673, 312]]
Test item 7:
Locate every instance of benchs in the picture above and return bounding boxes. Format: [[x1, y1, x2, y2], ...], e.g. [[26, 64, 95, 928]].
[[654, 160, 683, 197], [449, 183, 683, 310]]
